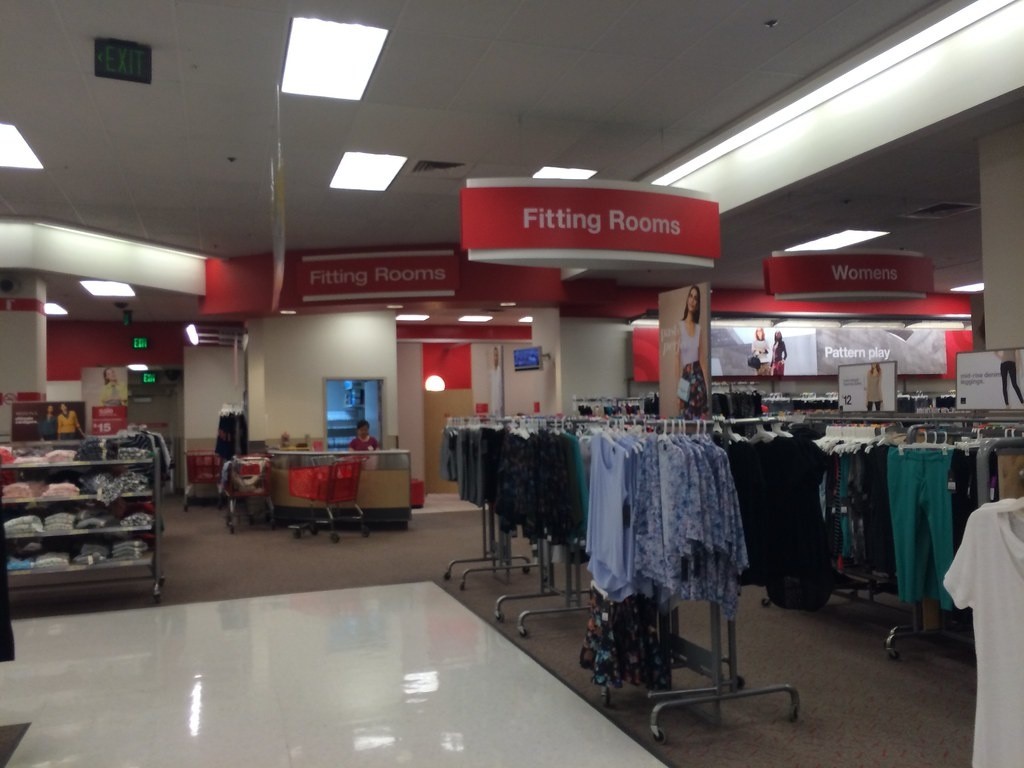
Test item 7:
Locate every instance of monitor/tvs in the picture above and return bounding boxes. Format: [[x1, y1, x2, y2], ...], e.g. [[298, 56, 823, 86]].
[[514, 347, 543, 371]]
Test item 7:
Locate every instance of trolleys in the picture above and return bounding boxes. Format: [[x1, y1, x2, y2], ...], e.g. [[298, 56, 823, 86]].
[[226, 453, 278, 534], [183, 449, 223, 512], [288, 454, 370, 543]]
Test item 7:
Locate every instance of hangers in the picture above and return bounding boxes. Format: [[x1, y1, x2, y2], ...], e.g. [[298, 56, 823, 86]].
[[442, 386, 1017, 456], [218, 403, 244, 417]]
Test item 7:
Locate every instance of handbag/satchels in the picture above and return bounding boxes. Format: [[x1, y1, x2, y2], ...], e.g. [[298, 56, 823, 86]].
[[677, 378, 690, 403], [747, 350, 762, 369]]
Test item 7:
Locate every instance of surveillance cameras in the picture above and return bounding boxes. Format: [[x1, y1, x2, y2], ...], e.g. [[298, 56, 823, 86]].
[[115, 302, 127, 309]]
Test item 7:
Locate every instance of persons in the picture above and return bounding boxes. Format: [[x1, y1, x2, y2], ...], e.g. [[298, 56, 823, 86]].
[[675, 286, 712, 420], [349, 419, 380, 452], [995, 349, 1024, 410], [98, 368, 128, 407], [491, 346, 503, 418], [752, 326, 788, 376], [38, 402, 85, 441], [866, 362, 883, 411]]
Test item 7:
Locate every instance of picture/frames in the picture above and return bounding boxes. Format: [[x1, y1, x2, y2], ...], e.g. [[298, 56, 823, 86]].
[[955, 347, 1024, 412], [837, 361, 897, 412]]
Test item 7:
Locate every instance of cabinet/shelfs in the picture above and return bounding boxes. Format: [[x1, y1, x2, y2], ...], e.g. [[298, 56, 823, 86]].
[[0, 433, 166, 606]]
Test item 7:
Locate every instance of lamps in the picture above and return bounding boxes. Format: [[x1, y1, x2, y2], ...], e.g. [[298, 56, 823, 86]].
[[186, 324, 249, 350]]
